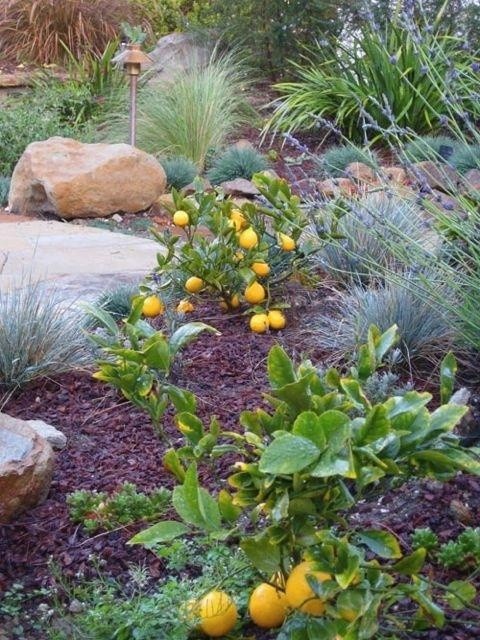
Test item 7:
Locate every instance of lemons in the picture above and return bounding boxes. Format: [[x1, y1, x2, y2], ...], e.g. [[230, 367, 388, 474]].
[[142, 208, 296, 333], [179, 560, 359, 637]]
